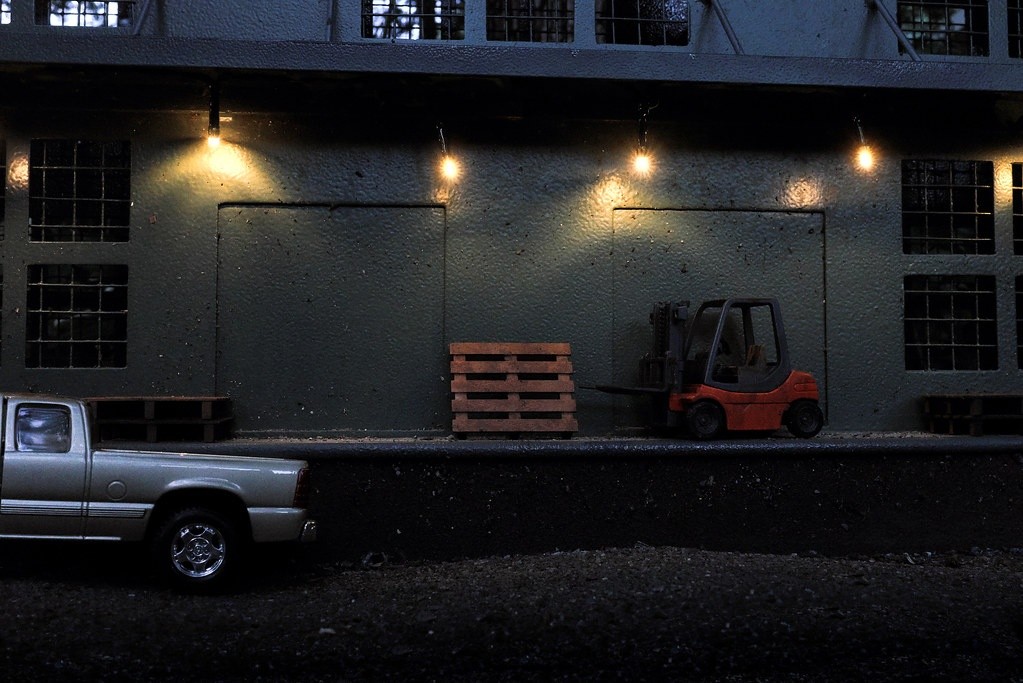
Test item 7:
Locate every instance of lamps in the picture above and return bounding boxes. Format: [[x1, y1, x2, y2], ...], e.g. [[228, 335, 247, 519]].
[[854, 119, 876, 171], [438, 126, 457, 179], [207, 94, 220, 148], [634, 119, 650, 173]]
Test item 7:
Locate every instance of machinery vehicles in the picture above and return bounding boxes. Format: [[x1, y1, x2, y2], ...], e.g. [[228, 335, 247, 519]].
[[577, 295, 825, 440]]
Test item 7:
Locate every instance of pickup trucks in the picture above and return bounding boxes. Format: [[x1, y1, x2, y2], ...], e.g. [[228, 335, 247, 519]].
[[1, 393, 318, 583]]
